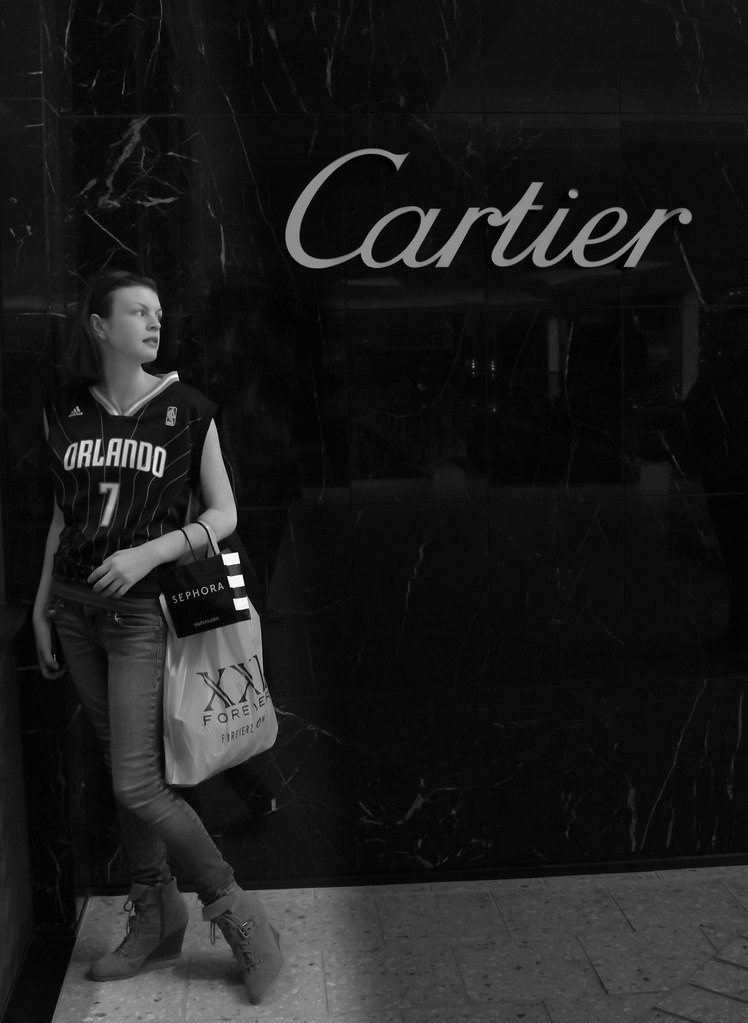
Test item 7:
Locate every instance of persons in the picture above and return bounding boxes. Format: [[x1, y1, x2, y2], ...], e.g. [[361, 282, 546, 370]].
[[33, 272, 281, 1002], [683, 305, 748, 651]]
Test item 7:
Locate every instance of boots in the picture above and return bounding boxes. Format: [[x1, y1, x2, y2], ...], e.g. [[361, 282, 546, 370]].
[[201, 886, 283, 1004], [88, 876, 189, 982]]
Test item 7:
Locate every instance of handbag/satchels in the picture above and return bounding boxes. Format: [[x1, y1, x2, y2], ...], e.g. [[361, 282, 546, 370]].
[[157, 521, 251, 638], [163, 519, 279, 788]]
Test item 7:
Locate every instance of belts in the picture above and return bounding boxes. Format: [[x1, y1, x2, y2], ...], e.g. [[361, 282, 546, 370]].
[[59, 597, 115, 619]]
